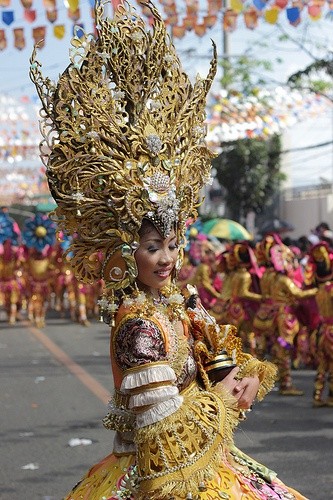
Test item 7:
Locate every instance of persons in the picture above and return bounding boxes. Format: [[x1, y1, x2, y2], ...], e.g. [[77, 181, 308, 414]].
[[174, 214, 333, 409], [62, 203, 310, 500], [0, 211, 108, 330]]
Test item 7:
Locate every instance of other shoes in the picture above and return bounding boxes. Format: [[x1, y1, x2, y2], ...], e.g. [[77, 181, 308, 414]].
[[313, 398, 333, 407], [279, 389, 304, 396]]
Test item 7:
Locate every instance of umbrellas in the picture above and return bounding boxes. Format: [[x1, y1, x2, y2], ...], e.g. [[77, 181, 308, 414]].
[[193, 217, 254, 243]]
